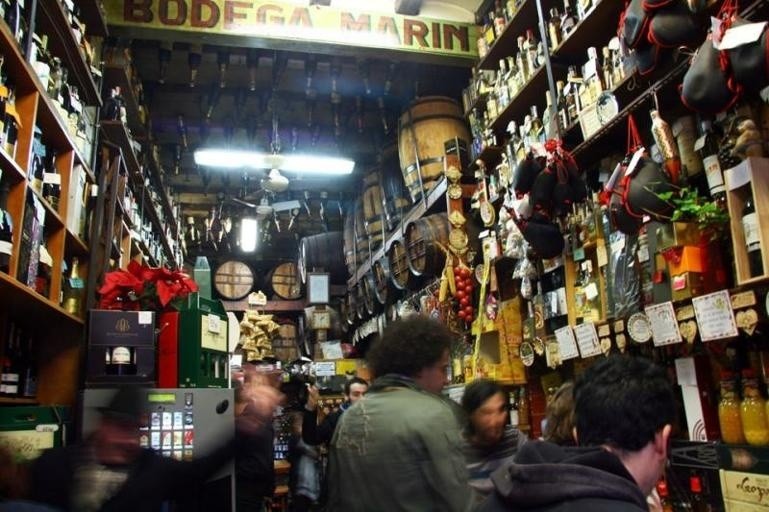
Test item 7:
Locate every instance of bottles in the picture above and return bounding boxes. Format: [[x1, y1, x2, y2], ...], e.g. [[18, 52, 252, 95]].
[[0, 0, 769, 512]]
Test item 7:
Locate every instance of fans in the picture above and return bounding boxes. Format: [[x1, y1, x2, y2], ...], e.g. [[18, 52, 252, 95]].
[[232, 112, 335, 222]]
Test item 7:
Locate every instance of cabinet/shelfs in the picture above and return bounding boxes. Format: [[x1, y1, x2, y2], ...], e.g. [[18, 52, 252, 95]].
[[342, 1, 769, 475], [0, 0, 187, 406]]
